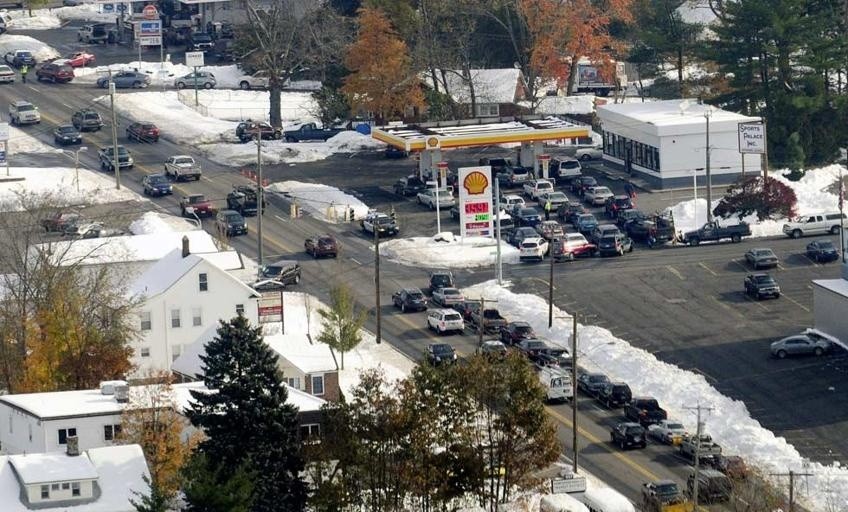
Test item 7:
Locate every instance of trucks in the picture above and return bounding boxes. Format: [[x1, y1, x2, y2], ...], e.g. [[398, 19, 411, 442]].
[[164, 154, 203, 182], [544, 56, 627, 98]]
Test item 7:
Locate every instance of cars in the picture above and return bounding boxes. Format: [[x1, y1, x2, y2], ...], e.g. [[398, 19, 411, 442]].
[[175, 72, 216, 88], [359, 210, 400, 237], [52, 51, 95, 65], [142, 173, 174, 197], [180, 194, 213, 218], [97, 71, 151, 88], [744, 247, 778, 268], [55, 125, 82, 145], [4, 49, 36, 69], [769, 334, 832, 358], [806, 239, 838, 262], [0, 64, 15, 83], [41, 211, 104, 240], [393, 145, 675, 264], [0, 12, 12, 32], [62, 0, 95, 6]]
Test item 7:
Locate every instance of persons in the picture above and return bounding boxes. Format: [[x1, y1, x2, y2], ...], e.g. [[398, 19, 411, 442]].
[[21, 62, 28, 82]]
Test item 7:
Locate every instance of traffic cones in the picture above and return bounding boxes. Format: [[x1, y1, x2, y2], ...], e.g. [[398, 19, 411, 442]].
[[239, 166, 267, 186]]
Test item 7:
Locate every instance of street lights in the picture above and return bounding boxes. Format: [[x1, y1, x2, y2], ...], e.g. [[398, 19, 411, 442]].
[[211, 7, 227, 20], [257, 148, 269, 277], [703, 108, 716, 224]]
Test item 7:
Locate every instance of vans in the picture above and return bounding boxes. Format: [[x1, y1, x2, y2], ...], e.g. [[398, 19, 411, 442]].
[[257, 260, 302, 290]]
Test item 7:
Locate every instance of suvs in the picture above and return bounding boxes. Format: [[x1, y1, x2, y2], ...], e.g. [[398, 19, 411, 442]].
[[237, 69, 291, 90], [126, 120, 159, 143], [214, 210, 248, 236], [77, 23, 108, 45], [186, 33, 216, 52], [305, 234, 338, 259], [72, 108, 103, 131], [36, 63, 75, 84], [237, 120, 282, 141], [227, 185, 265, 216], [744, 274, 782, 301], [8, 100, 40, 125]]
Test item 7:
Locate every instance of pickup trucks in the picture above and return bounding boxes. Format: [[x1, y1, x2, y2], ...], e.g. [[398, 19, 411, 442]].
[[283, 122, 339, 142], [683, 220, 752, 246], [781, 210, 848, 238], [97, 145, 134, 172]]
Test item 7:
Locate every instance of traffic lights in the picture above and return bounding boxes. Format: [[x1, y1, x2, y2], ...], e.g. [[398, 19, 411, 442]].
[[296, 204, 303, 218], [390, 209, 396, 225], [349, 208, 355, 222], [343, 208, 348, 222]]
